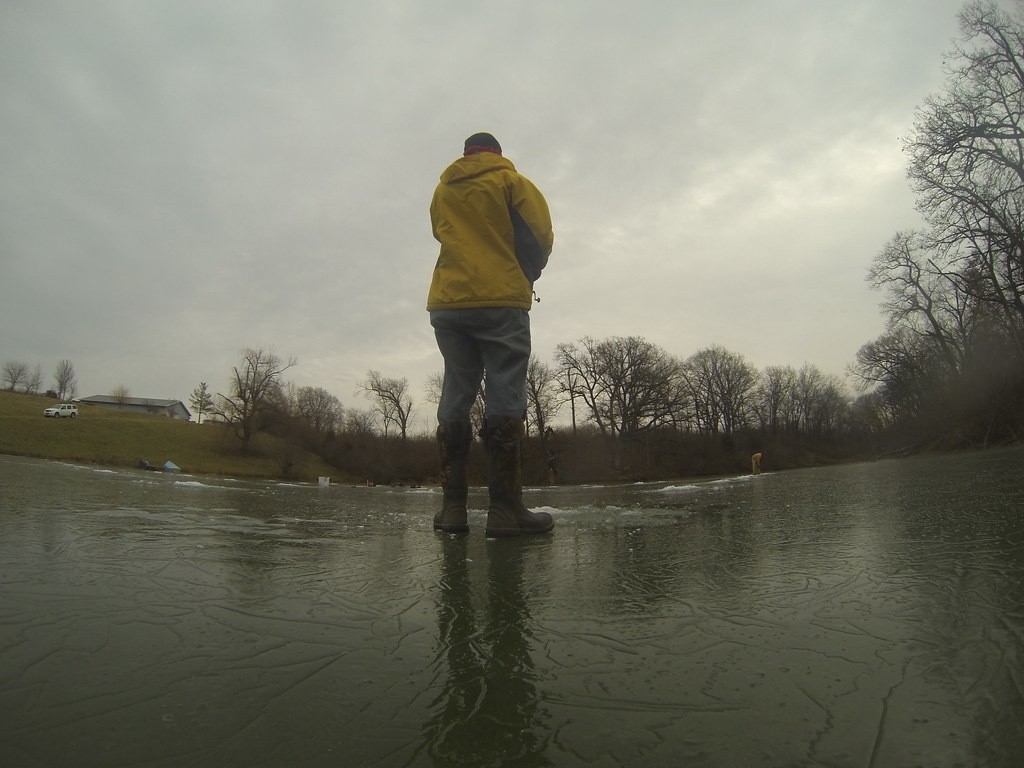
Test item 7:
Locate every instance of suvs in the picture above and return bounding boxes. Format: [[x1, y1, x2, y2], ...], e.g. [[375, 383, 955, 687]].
[[44, 403, 80, 419]]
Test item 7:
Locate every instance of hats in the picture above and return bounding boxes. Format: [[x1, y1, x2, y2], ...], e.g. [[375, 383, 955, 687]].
[[463, 132, 502, 156]]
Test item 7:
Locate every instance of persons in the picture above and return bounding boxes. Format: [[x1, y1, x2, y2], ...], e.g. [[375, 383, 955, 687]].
[[751, 452, 767, 475], [426, 132, 555, 534]]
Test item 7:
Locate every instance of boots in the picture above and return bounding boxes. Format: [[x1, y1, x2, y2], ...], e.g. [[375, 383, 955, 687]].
[[481, 415, 555, 538], [434, 421, 473, 533]]
